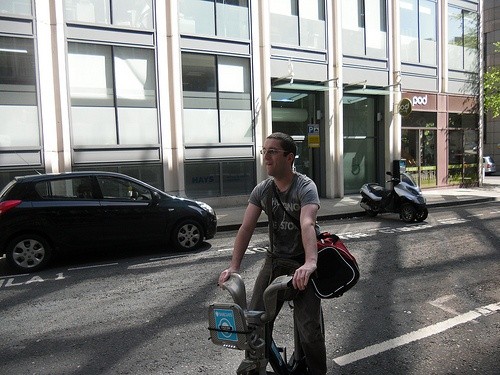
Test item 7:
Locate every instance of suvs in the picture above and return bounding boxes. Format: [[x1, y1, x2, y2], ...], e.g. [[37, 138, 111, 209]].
[[483, 156, 496, 175]]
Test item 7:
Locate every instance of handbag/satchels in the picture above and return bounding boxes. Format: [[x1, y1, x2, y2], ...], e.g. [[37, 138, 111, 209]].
[[309, 232, 360, 298]]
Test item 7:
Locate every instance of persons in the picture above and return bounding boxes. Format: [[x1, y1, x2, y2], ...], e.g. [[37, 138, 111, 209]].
[[218, 132, 330, 374]]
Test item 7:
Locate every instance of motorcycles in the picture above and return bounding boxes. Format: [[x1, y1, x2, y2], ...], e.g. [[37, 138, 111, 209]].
[[359, 170, 427, 223]]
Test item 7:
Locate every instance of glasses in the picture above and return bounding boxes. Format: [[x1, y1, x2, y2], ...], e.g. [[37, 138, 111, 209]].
[[260, 149, 290, 156]]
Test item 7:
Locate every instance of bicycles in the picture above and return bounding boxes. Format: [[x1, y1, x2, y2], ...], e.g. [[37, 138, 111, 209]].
[[207, 271, 325, 374]]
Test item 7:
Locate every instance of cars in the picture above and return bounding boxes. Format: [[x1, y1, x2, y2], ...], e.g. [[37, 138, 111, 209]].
[[0, 171, 217, 274]]
